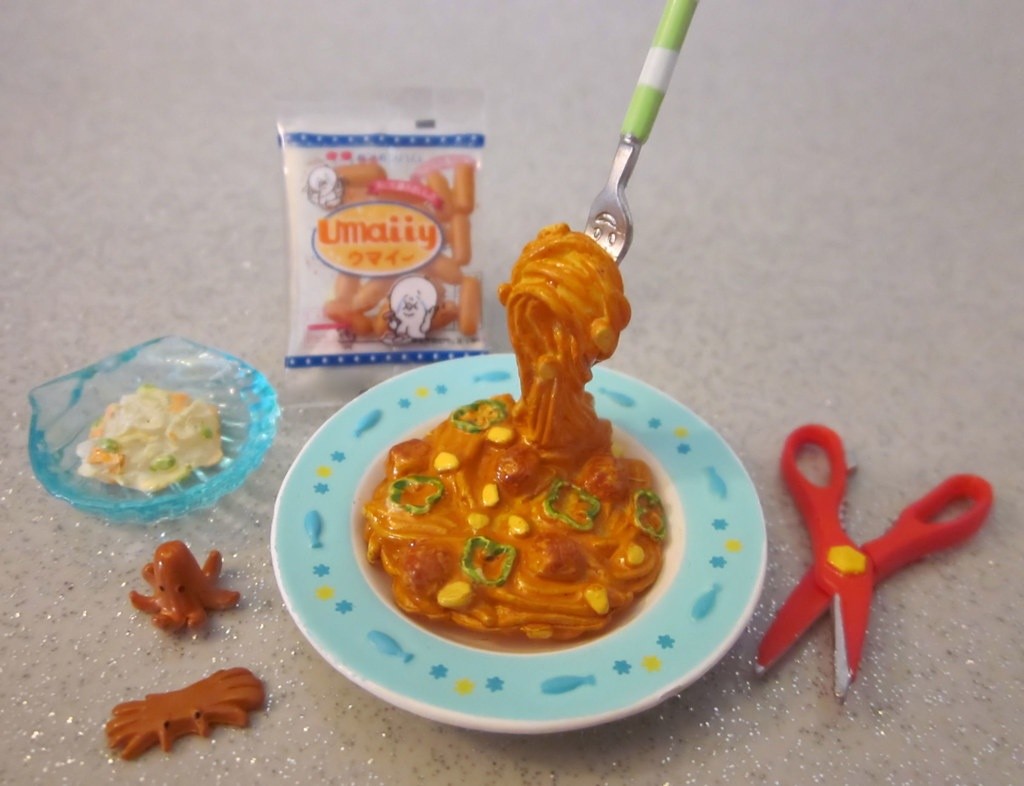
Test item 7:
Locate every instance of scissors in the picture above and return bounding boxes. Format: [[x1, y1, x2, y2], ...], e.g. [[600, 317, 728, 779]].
[[753, 421, 997, 705]]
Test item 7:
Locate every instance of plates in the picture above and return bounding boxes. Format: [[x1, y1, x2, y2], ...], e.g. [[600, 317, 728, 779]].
[[25, 335, 280, 523], [272, 351, 768, 732]]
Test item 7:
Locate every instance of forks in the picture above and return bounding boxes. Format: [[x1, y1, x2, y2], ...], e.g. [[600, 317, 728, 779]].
[[584, 0, 696, 263]]
